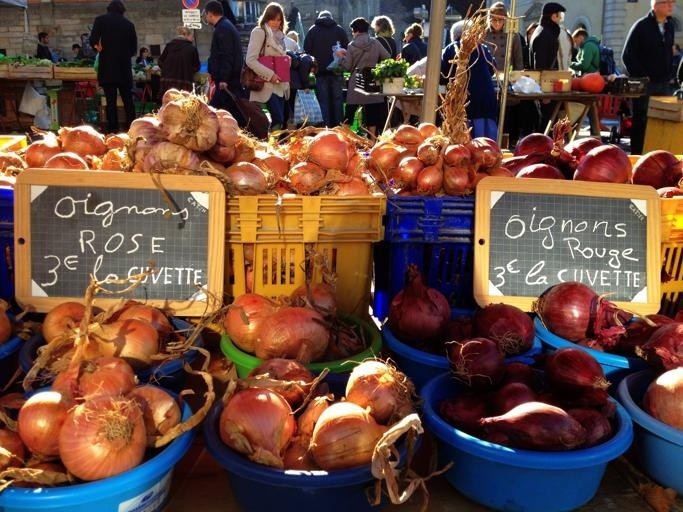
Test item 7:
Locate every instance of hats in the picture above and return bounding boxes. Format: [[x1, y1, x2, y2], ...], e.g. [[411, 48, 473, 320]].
[[542, 2, 566, 15]]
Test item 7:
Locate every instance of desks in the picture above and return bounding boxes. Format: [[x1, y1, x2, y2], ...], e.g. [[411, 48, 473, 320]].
[[498, 89, 645, 145], [640, 95, 683, 155], [388, 86, 447, 126]]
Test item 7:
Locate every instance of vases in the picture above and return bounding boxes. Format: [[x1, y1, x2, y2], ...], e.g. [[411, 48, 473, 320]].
[[383, 77, 405, 96]]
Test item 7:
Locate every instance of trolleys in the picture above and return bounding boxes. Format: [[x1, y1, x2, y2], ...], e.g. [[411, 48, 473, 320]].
[[222, 86, 272, 140]]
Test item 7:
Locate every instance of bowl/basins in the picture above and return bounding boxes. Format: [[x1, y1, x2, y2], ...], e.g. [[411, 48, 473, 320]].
[[382, 311, 541, 380], [535, 308, 647, 374], [619, 370, 683, 491], [0, 386, 195, 511], [19, 316, 202, 385], [0, 335, 20, 362], [220, 312, 381, 376], [204, 396, 419, 511], [419, 362, 633, 511]]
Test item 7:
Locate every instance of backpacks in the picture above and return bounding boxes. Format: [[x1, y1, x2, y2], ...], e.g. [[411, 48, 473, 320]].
[[595, 41, 615, 76]]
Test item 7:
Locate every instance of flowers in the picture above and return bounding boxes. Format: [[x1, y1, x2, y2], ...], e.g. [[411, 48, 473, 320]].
[[371, 54, 409, 83]]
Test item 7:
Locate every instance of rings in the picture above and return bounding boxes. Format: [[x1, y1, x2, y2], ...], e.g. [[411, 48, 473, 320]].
[[276, 80, 279, 84]]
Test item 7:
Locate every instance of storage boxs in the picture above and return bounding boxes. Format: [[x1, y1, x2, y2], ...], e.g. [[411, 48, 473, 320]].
[[54, 62, 98, 81], [509, 70, 541, 89], [541, 70, 572, 93], [8, 62, 54, 80], [0, 64, 8, 79]]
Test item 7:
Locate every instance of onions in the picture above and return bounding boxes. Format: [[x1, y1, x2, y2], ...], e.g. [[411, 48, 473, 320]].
[[1, 297, 189, 492], [534, 282, 683, 371], [219, 278, 417, 470], [225, 130, 369, 294], [504, 132, 683, 199], [437, 338, 619, 452], [0, 91, 239, 190], [390, 278, 536, 358], [368, 122, 513, 197], [642, 367, 683, 431]]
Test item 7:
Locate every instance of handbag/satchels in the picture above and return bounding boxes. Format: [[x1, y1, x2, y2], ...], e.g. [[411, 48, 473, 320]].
[[293, 88, 324, 125], [252, 55, 292, 82], [239, 58, 264, 92]]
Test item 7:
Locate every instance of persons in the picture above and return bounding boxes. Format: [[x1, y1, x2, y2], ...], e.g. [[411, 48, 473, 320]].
[[567, 31, 578, 62], [401, 23, 428, 63], [481, 2, 524, 70], [278, 54, 319, 144], [574, 23, 586, 30], [36, 31, 54, 62], [568, 27, 603, 73], [669, 43, 682, 97], [245, 2, 293, 130], [287, 30, 300, 43], [157, 26, 201, 104], [439, 18, 499, 142], [89, 0, 138, 132], [136, 46, 153, 88], [334, 16, 392, 126], [620, 0, 676, 156], [71, 43, 83, 60], [525, 22, 540, 46], [204, 1, 244, 108], [528, 2, 572, 136], [303, 9, 349, 128], [370, 15, 397, 57]]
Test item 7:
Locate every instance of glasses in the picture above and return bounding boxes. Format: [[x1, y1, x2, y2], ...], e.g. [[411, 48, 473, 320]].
[[489, 19, 505, 24]]
